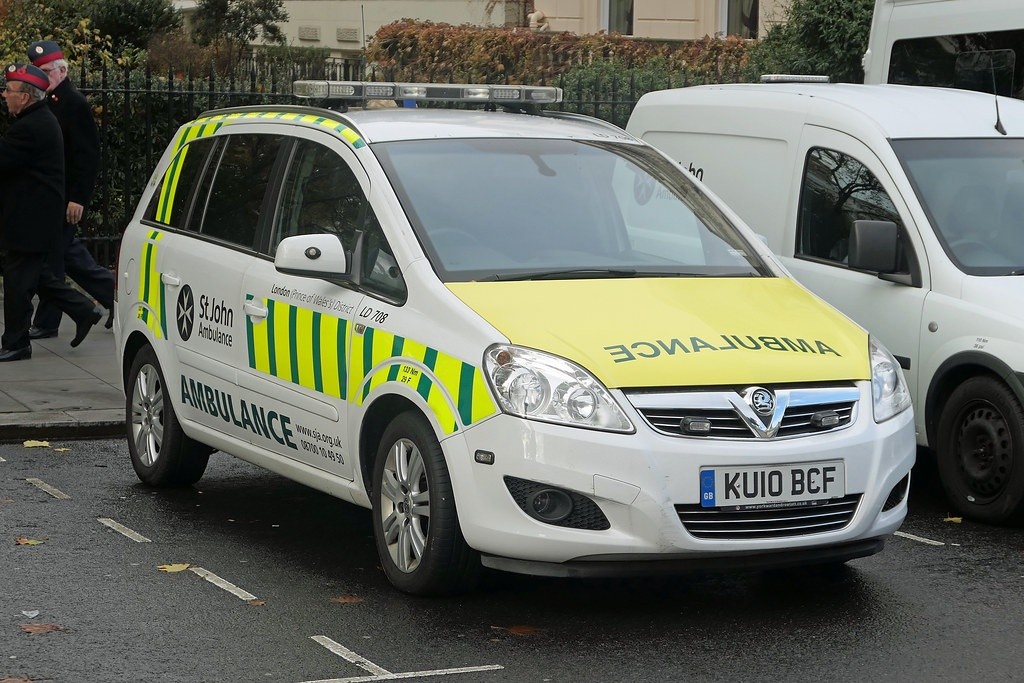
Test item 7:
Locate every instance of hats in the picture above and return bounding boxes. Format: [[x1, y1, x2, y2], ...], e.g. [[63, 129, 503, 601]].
[[5, 64, 51, 92], [26, 40, 64, 67]]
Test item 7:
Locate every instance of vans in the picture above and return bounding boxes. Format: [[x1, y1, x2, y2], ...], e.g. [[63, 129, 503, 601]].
[[862, 0, 1024, 103], [609, 79, 1024, 527]]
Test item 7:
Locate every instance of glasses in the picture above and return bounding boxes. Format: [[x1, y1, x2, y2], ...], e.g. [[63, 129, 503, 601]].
[[41, 65, 62, 74], [4, 86, 31, 99]]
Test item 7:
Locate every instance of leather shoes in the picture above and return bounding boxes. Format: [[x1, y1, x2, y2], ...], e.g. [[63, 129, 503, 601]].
[[1, 345, 32, 362], [69, 306, 103, 348], [29, 324, 59, 339], [105, 307, 114, 328]]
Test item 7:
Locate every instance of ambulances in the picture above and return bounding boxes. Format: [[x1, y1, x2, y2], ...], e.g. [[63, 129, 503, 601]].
[[112, 76, 920, 615]]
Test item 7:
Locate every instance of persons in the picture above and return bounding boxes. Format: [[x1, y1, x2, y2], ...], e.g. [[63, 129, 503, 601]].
[[23, 40, 122, 338], [0, 63, 103, 362]]
